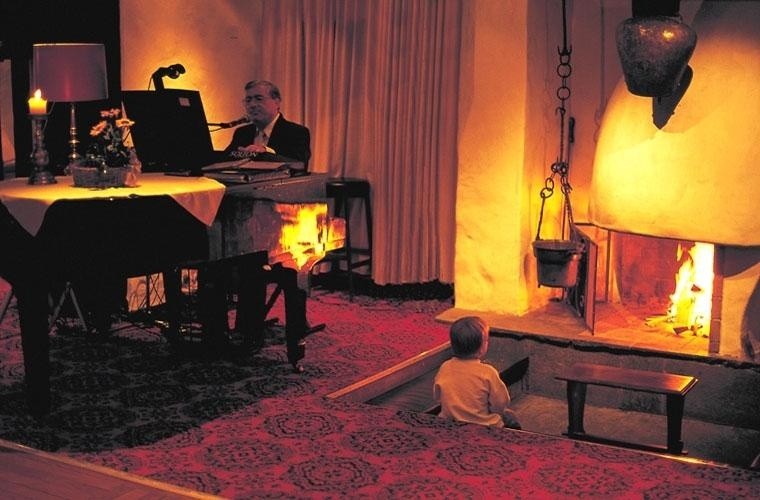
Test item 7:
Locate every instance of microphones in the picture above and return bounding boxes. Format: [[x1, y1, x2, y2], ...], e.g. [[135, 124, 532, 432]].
[[221, 113, 252, 128]]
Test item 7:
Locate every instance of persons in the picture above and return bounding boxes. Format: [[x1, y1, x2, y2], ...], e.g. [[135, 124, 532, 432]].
[[432, 317, 521, 431], [196, 78, 311, 362]]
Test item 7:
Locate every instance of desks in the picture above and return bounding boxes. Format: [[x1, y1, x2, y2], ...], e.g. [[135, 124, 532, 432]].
[[555, 359, 700, 455]]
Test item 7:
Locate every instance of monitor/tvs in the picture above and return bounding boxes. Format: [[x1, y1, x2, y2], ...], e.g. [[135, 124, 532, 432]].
[[120, 87, 215, 174]]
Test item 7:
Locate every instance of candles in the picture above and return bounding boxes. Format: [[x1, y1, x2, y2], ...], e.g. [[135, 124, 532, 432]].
[[28, 91, 47, 115]]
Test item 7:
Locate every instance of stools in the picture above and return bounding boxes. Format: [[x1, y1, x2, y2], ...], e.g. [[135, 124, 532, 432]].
[[310, 176, 373, 304]]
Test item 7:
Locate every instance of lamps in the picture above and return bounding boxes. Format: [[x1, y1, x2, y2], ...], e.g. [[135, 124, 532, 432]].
[[31, 41, 108, 160]]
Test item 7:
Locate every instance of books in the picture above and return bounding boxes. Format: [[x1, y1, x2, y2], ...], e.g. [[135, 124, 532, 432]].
[[201, 158, 291, 184]]
